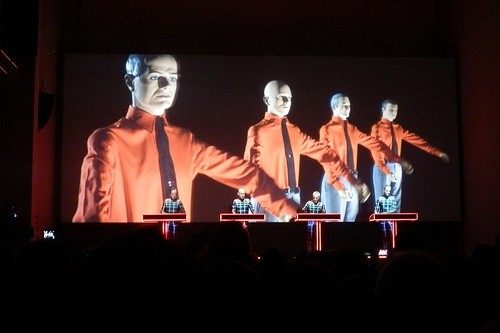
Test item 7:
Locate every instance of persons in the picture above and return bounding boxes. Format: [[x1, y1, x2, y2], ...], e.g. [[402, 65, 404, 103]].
[[162, 186, 187, 213], [230, 187, 255, 214], [319, 93, 414, 221], [370, 100, 453, 249], [376, 185, 396, 235], [71, 52, 301, 222], [244, 81, 369, 207], [301, 190, 327, 251]]
